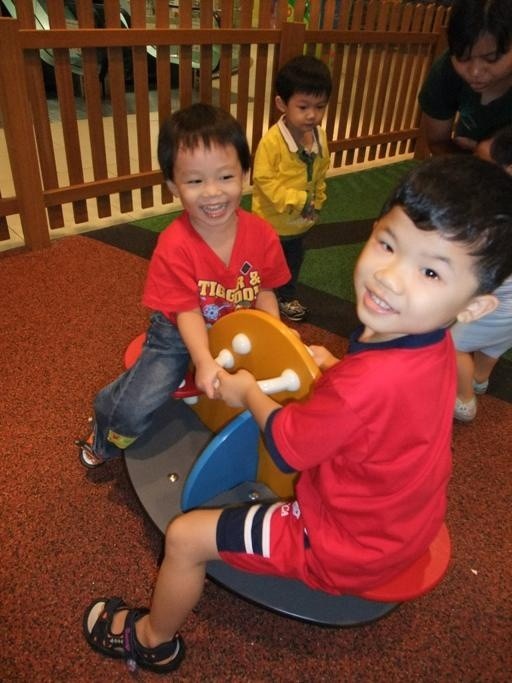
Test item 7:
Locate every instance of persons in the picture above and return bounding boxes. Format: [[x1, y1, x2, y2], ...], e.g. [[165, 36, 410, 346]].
[[449, 124, 511, 422], [251, 55, 333, 322], [416, 0, 511, 166], [79, 103, 293, 471], [84, 153, 511, 674]]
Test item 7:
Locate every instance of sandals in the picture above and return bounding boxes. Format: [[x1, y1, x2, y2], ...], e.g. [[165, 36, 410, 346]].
[[74, 427, 108, 470], [82, 594, 185, 677]]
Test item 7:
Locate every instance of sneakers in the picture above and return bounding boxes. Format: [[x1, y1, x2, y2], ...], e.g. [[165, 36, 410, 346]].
[[453, 379, 490, 424], [276, 298, 310, 322]]
